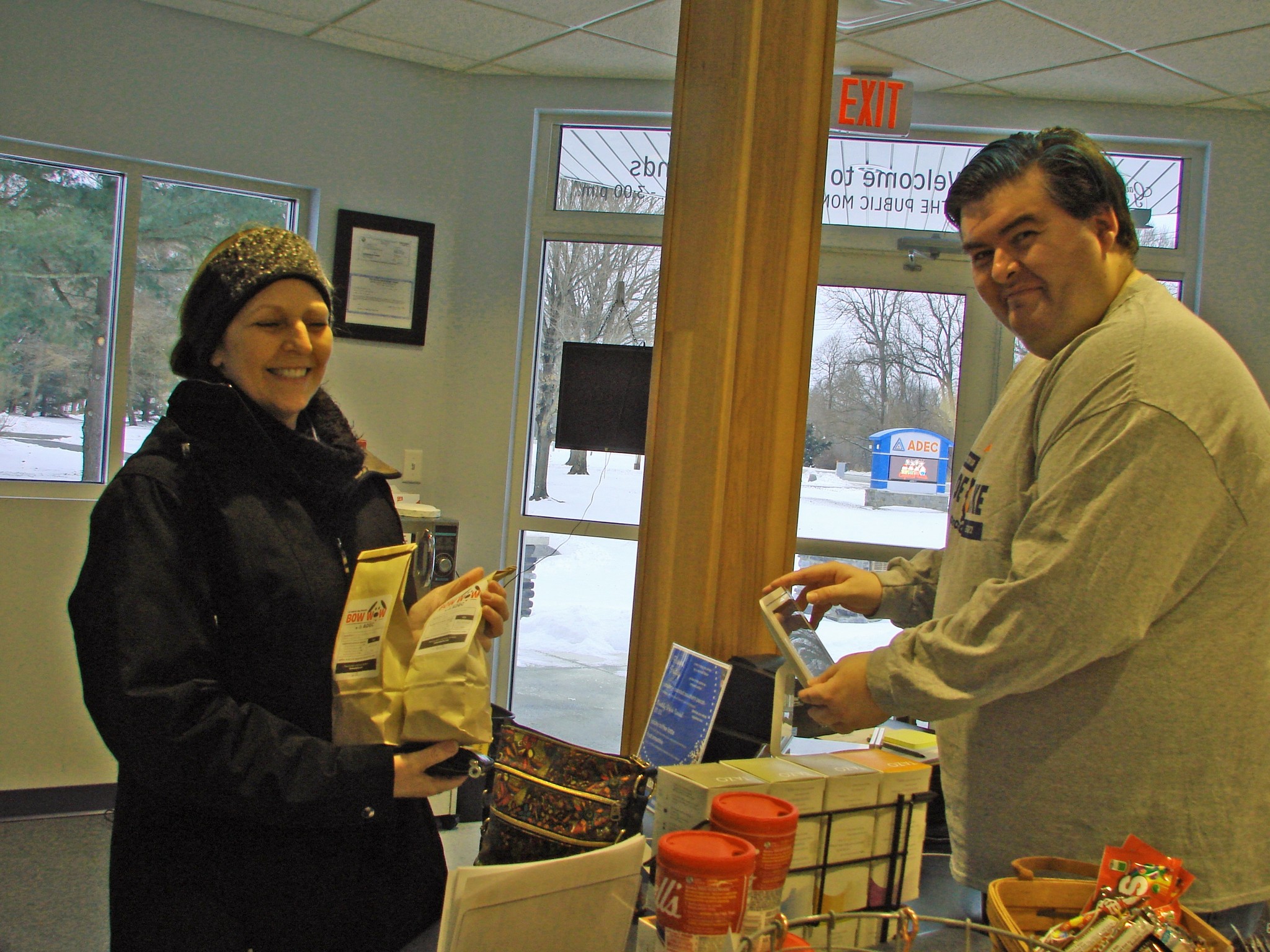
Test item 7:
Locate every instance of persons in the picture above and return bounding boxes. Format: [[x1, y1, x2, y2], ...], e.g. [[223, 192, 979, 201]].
[[762, 125, 1270, 952], [65, 224, 507, 952]]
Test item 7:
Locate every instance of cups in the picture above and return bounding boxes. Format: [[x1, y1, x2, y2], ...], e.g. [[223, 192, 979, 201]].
[[710, 791, 799, 952], [655, 830, 756, 952], [783, 933, 816, 952]]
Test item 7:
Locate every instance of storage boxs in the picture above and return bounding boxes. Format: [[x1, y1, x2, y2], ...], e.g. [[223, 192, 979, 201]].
[[636, 748, 935, 952]]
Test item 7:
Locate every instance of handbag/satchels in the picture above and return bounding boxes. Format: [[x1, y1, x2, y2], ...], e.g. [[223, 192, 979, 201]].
[[475, 722, 653, 867]]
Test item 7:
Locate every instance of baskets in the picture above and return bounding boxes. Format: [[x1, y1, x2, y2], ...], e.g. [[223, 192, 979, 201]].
[[986, 855, 1234, 952]]
